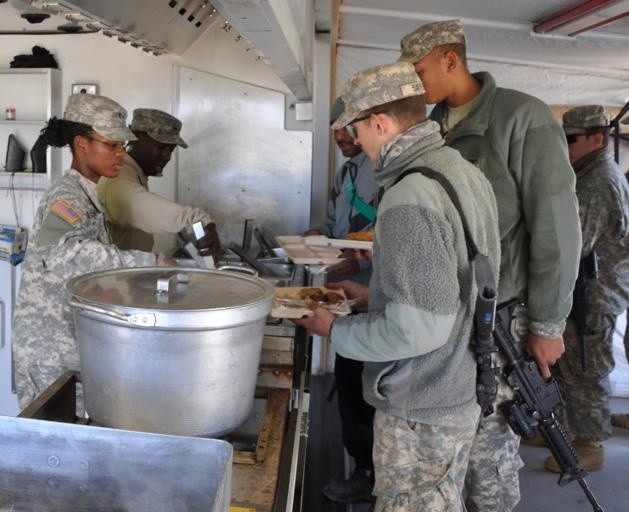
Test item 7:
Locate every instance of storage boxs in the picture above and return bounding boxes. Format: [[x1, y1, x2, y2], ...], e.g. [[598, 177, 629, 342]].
[[0, 226, 28, 266]]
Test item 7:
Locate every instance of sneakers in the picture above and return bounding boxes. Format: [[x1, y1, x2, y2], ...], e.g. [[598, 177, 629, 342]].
[[323, 468, 375, 504], [545, 441, 604, 473], [521, 424, 575, 446], [611, 413, 629, 429]]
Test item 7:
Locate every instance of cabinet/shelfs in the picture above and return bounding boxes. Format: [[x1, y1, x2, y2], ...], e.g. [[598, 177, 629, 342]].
[[0, 65, 59, 190]]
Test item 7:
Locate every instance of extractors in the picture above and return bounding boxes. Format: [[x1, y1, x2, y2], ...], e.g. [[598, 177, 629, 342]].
[[34, 1, 315, 104]]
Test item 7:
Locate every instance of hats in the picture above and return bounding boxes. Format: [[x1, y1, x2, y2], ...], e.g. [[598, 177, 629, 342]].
[[63, 93, 139, 142], [330, 97, 345, 124], [131, 108, 188, 149], [397, 20, 465, 64], [562, 105, 607, 135], [330, 61, 426, 131]]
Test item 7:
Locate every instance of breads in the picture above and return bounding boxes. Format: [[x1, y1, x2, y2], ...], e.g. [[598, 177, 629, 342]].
[[345, 231, 374, 241], [299, 287, 344, 304]]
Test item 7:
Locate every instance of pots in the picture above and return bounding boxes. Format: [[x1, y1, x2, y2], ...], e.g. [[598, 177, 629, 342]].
[[66, 264, 276, 441]]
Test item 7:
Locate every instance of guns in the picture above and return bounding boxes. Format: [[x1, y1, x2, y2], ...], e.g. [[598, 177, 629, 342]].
[[472, 285, 503, 417], [572, 250, 598, 372], [495, 296, 602, 512]]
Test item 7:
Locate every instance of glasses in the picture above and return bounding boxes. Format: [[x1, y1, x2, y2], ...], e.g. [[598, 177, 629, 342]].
[[345, 109, 390, 139], [87, 135, 134, 153], [567, 131, 602, 145]]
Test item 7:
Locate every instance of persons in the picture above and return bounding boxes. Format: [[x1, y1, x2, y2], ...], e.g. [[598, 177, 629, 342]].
[[96, 109, 220, 268], [12, 94, 181, 412]]
